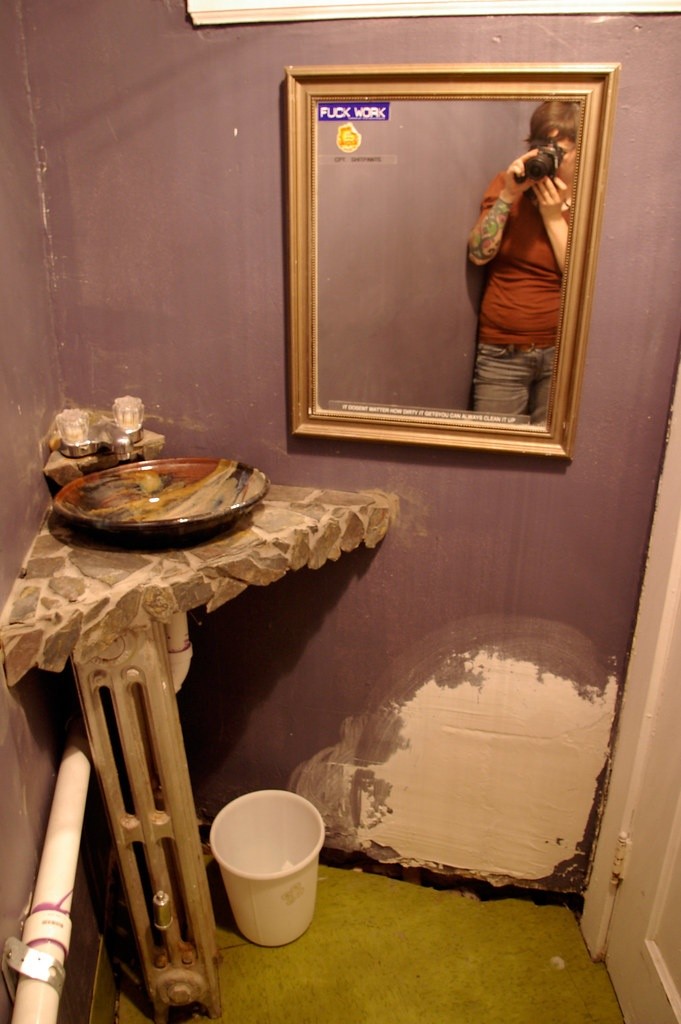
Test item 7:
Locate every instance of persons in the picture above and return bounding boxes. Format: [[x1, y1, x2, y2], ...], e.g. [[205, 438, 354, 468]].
[[467, 101, 583, 426]]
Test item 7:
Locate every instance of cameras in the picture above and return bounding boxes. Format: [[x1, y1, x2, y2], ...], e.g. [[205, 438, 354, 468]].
[[524, 142, 564, 182]]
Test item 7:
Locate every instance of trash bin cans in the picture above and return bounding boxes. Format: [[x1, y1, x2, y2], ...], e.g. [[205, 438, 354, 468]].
[[209, 789, 325, 948]]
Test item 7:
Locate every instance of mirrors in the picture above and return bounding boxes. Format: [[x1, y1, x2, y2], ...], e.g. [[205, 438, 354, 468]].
[[284, 62, 619, 462]]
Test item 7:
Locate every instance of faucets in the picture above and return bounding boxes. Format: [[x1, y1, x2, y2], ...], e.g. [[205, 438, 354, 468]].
[[98, 420, 133, 464]]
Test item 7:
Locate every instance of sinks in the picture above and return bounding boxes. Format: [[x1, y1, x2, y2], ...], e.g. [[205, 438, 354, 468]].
[[52, 451, 271, 541]]
